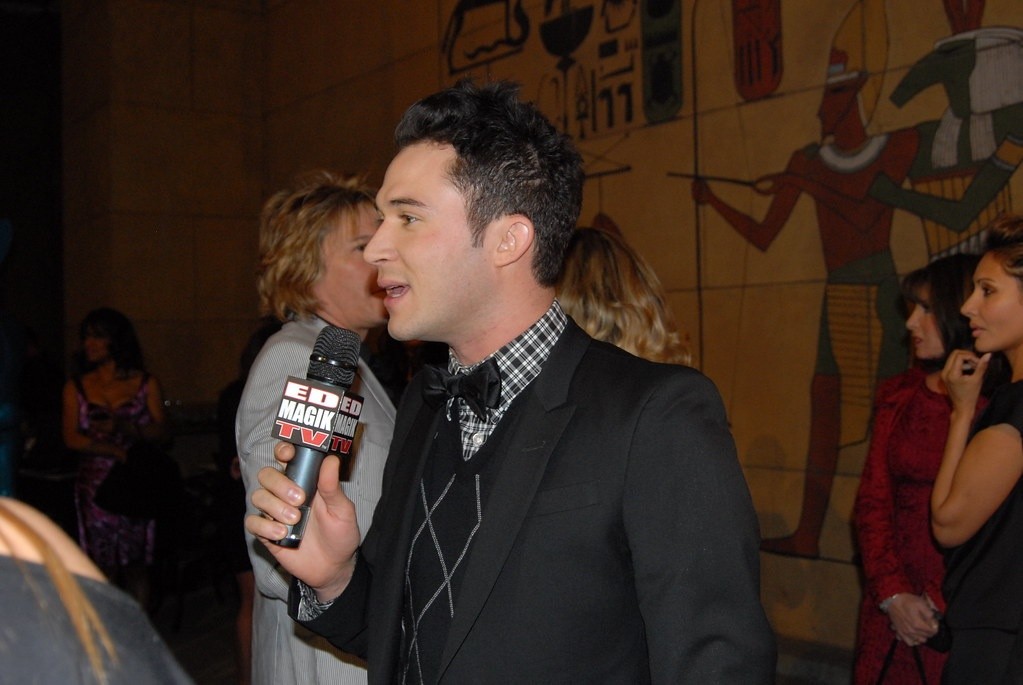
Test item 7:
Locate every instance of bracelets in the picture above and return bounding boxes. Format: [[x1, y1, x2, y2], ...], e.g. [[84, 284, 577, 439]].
[[878, 593, 897, 614]]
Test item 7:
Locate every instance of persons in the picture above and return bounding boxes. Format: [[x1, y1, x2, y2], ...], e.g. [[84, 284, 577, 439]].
[[854, 251, 1012, 684], [60, 307, 164, 604], [244, 74, 779, 685], [554, 226, 697, 366], [0, 494, 194, 685], [930, 213, 1023, 685], [235, 170, 398, 685]]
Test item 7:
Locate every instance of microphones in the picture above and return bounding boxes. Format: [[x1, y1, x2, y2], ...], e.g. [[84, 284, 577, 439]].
[[270, 324, 364, 549]]
